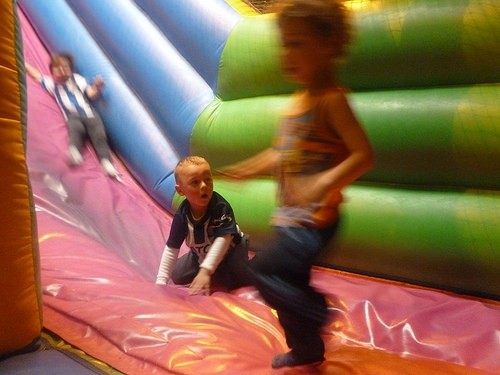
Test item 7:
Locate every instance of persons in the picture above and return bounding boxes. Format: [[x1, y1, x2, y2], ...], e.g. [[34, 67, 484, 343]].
[[24, 52, 120, 177], [217, 0, 377, 370], [156, 156, 260, 296]]
